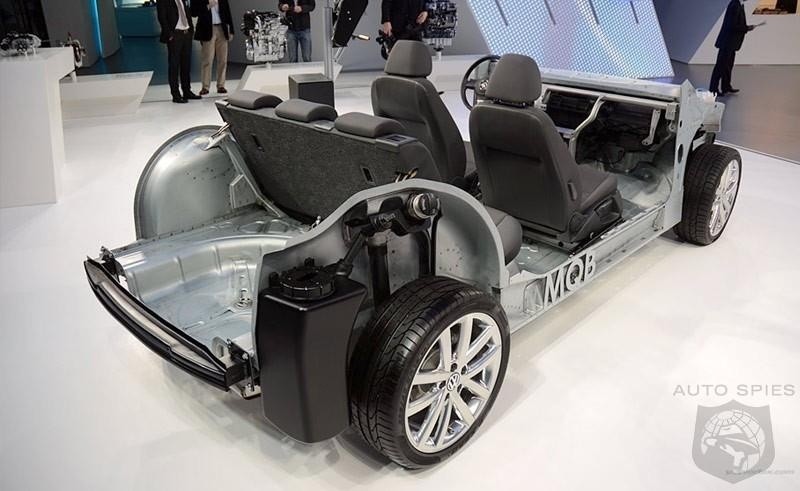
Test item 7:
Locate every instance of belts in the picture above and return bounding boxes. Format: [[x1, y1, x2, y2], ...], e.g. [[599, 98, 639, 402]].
[[183, 30, 189, 34]]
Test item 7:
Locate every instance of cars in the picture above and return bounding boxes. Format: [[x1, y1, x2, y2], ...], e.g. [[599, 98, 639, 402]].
[[81, 38, 744, 470]]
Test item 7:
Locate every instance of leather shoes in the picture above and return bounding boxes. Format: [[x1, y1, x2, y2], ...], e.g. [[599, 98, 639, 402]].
[[173, 97, 188, 104], [709, 89, 724, 97], [218, 88, 226, 93], [722, 88, 740, 94], [200, 89, 209, 95], [184, 91, 201, 99]]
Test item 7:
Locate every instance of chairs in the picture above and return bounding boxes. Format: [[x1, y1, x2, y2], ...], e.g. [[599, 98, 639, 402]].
[[370, 38, 482, 190], [275, 97, 337, 123], [225, 88, 280, 109], [466, 52, 624, 252], [335, 110, 527, 266]]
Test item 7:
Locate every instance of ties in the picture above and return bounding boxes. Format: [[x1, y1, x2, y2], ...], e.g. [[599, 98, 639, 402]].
[[177, 1, 186, 27]]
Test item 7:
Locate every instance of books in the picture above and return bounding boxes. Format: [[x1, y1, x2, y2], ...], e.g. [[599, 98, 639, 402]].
[[226, 23, 231, 37]]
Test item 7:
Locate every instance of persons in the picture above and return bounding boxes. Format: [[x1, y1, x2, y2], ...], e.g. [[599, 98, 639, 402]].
[[155, 0, 202, 103], [710, 0, 754, 96], [278, 0, 315, 63], [380, 1, 429, 54], [189, 0, 234, 94]]
